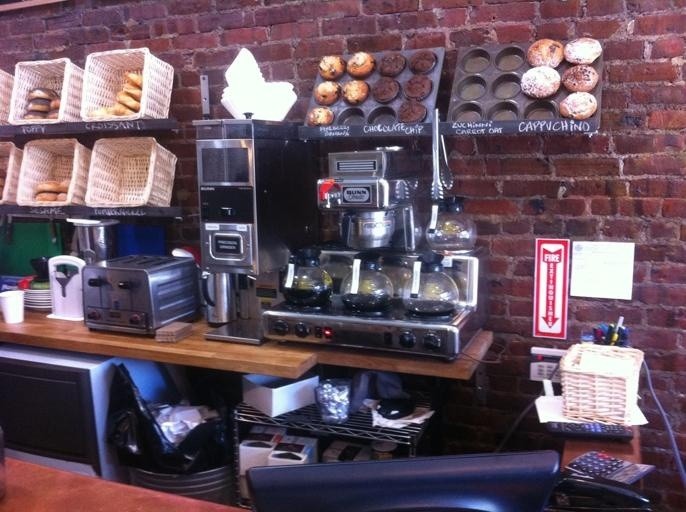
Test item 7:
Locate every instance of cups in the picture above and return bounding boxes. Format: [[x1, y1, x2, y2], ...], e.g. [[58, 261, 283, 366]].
[[315, 378, 350, 426], [0, 291, 27, 324]]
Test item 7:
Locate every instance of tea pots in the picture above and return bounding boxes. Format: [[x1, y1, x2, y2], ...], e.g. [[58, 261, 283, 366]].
[[280, 195, 478, 315]]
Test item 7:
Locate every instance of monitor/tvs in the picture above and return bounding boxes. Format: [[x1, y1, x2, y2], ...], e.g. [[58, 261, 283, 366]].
[[246, 449, 559, 512]]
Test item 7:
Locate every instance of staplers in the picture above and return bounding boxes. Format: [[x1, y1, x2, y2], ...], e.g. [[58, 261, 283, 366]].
[[545, 477, 652, 511]]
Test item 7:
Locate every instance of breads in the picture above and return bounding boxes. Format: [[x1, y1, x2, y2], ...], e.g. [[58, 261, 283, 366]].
[[519, 35, 603, 119], [308, 48, 438, 129], [1, 171, 7, 200], [34, 179, 70, 203], [87, 72, 142, 118], [22, 86, 60, 123]]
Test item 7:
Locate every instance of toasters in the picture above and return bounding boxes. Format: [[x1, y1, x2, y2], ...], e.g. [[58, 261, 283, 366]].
[[82, 254, 199, 339]]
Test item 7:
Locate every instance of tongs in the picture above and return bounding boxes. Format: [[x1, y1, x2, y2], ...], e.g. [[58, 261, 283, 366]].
[[429, 106, 454, 200]]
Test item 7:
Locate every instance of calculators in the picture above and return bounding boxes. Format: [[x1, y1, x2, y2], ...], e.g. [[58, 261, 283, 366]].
[[564, 450, 656, 485]]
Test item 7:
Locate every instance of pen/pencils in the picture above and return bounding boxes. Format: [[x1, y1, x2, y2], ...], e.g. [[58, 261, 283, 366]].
[[594, 315, 629, 345]]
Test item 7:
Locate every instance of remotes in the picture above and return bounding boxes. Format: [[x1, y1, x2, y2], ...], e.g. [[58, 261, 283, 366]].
[[546, 420, 638, 443]]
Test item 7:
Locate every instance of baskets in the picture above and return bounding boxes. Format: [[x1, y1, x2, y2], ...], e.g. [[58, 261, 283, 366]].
[[0, 57, 84, 126], [85, 137, 179, 208], [0, 141, 23, 205], [558, 343, 645, 427], [81, 46, 176, 122], [16, 138, 92, 207]]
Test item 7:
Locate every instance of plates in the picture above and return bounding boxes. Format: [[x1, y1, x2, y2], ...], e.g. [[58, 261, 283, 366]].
[[23, 282, 52, 312]]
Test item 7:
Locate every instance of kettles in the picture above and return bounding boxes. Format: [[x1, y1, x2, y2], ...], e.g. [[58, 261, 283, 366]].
[[202, 270, 238, 329]]
